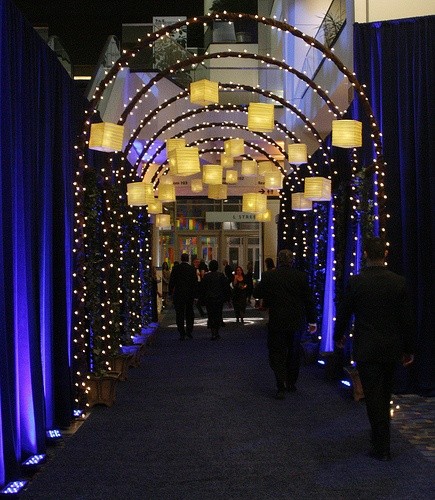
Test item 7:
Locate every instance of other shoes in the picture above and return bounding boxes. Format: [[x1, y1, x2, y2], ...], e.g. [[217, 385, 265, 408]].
[[286, 384, 297, 392], [272, 390, 284, 400], [368, 446, 392, 461]]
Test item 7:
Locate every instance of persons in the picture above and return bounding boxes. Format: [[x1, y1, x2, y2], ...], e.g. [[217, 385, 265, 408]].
[[255, 251, 320, 399], [162, 255, 276, 338], [342, 236, 416, 461]]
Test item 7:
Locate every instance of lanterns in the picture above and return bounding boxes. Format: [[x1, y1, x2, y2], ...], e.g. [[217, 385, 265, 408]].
[[248, 102, 274, 133], [332, 121, 362, 148], [127, 138, 332, 229], [89, 121, 124, 153], [191, 80, 218, 106]]
